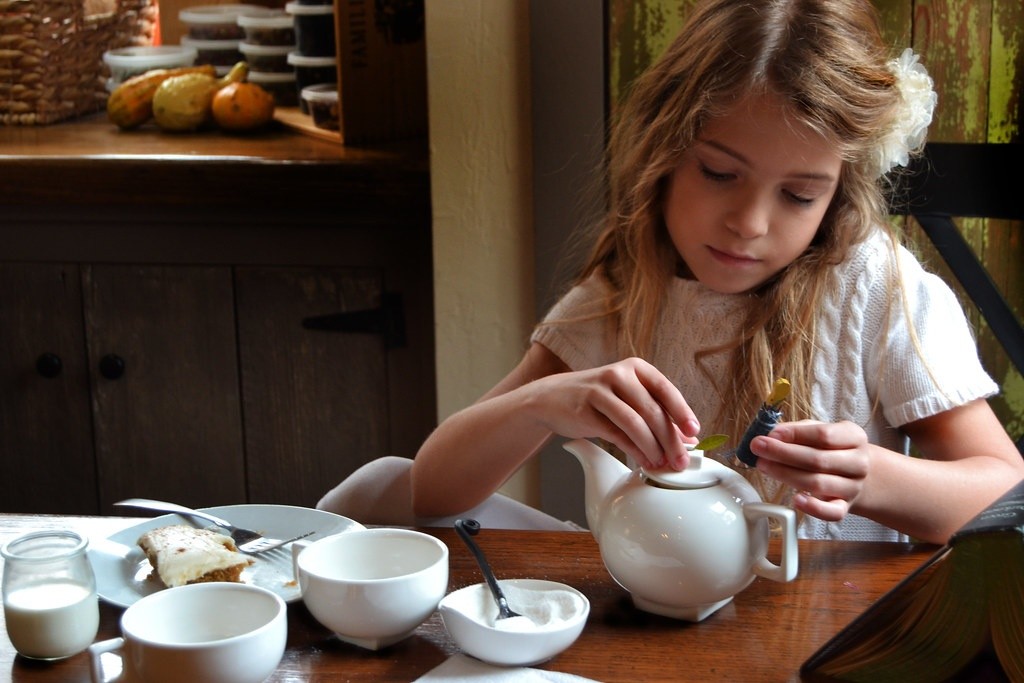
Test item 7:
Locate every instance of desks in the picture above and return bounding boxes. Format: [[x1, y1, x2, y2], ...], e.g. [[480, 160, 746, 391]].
[[0, 513, 942, 683], [0, 117, 435, 512]]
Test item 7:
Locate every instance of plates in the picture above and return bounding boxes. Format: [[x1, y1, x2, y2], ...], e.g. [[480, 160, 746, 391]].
[[88, 504, 367, 609]]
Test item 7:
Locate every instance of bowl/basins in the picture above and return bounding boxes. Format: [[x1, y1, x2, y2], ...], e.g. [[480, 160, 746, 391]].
[[438, 578, 591, 667]]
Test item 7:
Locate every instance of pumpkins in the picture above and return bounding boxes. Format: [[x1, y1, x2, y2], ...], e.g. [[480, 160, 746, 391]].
[[106, 60, 275, 133]]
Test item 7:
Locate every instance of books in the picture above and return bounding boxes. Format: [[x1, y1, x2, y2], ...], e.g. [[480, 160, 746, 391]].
[[800, 478, 1024, 683]]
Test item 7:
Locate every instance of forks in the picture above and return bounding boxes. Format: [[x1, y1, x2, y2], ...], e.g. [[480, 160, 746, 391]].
[[112, 499, 316, 554]]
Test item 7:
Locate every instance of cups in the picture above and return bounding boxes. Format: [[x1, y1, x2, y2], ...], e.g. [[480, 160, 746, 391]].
[[89, 582, 288, 683], [291, 527, 451, 650]]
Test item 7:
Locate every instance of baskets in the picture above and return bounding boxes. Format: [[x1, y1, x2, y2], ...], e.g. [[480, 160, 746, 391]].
[[0, 0, 158, 126]]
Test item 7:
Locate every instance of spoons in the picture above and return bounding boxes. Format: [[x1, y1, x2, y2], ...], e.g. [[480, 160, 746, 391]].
[[455, 517, 522, 622]]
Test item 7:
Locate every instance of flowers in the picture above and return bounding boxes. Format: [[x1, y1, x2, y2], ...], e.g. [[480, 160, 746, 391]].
[[876, 47, 938, 178]]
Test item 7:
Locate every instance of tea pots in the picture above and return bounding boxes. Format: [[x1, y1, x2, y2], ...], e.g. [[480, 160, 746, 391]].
[[561, 439, 798, 622]]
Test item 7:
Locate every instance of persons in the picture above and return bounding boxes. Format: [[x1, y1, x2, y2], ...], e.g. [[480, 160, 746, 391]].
[[314, 0, 1024, 544]]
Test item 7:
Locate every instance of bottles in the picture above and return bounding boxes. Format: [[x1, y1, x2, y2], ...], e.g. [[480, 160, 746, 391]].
[[0, 527, 100, 660]]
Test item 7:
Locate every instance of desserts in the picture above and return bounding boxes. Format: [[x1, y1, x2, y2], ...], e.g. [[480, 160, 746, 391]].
[[133, 526, 250, 588]]
